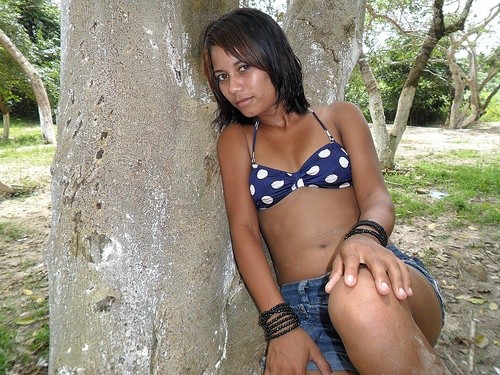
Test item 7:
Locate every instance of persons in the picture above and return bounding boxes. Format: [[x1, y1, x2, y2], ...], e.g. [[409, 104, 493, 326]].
[[199, 7, 457, 374]]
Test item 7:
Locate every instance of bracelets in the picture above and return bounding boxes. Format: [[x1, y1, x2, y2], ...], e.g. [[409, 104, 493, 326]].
[[343, 219, 388, 247], [257, 300, 301, 341]]
